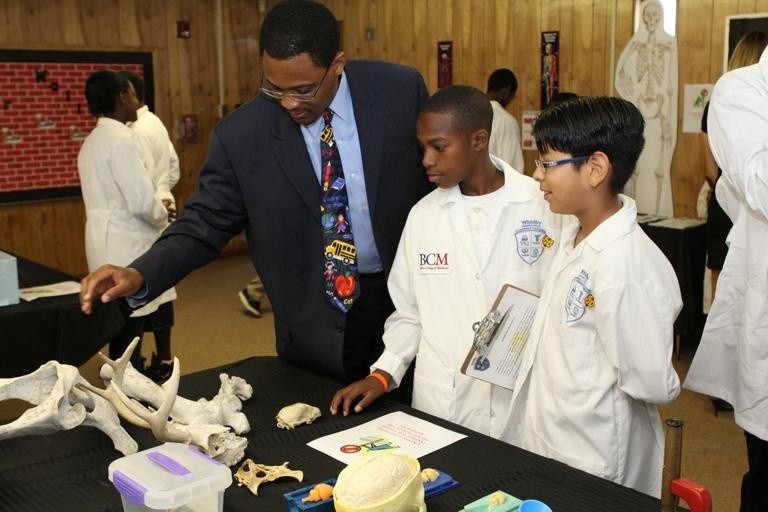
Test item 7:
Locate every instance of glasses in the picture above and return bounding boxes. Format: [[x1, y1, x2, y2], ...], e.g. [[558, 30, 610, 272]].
[[533, 157, 590, 171], [258, 67, 330, 104]]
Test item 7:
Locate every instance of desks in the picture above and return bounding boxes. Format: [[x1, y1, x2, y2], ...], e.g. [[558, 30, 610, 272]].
[[1, 352, 711, 512]]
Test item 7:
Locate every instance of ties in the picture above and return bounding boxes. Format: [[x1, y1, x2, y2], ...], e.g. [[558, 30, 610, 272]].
[[319, 105, 364, 314]]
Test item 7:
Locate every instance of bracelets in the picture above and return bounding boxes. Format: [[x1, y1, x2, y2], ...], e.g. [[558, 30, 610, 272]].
[[366, 370, 388, 393]]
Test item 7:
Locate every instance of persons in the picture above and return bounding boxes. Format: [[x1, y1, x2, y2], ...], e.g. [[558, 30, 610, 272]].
[[117, 70, 181, 365], [483, 67, 527, 176], [76, 68, 170, 373], [677, 44, 768, 512], [329, 85, 573, 450], [697, 33, 768, 415], [488, 92, 687, 499], [78, 2, 427, 413]]
